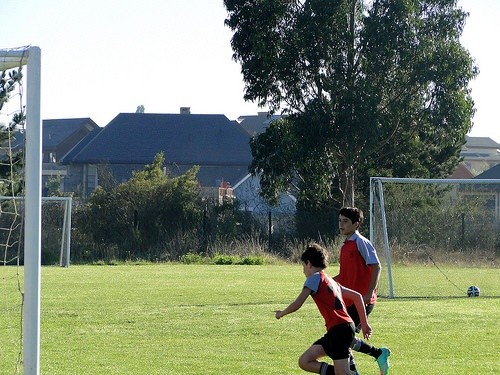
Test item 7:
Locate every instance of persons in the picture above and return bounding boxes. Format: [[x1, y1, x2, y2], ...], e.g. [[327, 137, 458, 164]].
[[331, 207, 390, 375], [274, 243, 371, 375]]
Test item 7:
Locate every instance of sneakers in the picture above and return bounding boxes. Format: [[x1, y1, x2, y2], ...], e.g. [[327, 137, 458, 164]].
[[375, 348, 390, 375]]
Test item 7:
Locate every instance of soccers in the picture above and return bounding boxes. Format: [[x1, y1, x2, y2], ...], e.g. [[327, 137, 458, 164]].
[[468, 286, 479, 296]]
